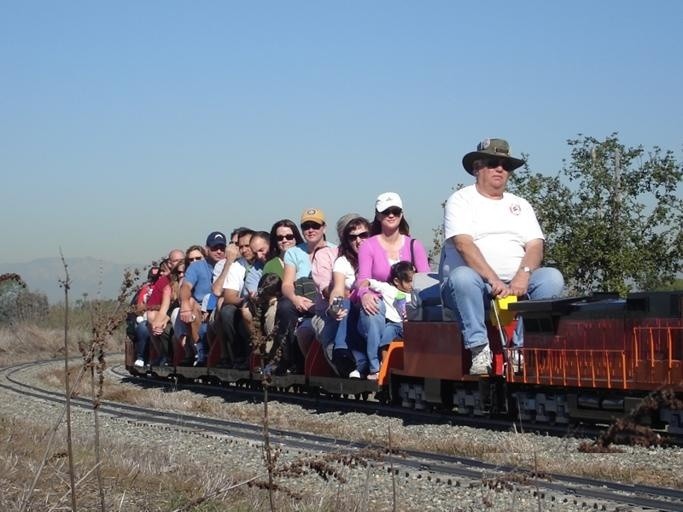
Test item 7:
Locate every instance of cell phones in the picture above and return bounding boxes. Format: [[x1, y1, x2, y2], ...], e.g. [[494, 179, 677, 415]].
[[191, 314, 196, 322]]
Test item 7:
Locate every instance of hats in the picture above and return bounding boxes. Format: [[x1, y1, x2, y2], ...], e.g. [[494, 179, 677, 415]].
[[300, 208, 325, 225], [375, 192, 402, 213], [462, 138, 524, 176], [337, 214, 359, 241], [206, 232, 226, 247]]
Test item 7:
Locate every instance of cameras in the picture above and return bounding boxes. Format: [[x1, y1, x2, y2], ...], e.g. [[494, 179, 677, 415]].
[[329, 295, 344, 315]]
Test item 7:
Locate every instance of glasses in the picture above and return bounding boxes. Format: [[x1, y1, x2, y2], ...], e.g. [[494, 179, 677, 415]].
[[301, 223, 320, 230], [210, 247, 224, 251], [188, 256, 201, 263], [381, 208, 400, 216], [348, 232, 368, 241], [276, 234, 294, 241], [484, 159, 513, 172]]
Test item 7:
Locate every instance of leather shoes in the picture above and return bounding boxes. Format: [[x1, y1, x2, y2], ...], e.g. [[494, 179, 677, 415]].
[[147, 352, 249, 370]]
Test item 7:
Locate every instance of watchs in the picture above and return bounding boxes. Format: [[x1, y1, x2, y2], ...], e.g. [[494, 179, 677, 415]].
[[518, 264, 533, 275]]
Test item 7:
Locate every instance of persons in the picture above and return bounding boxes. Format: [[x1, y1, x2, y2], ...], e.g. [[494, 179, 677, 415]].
[[441, 138, 565, 377], [124, 192, 433, 381]]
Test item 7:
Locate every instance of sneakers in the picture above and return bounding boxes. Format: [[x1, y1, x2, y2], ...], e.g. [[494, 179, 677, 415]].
[[133, 359, 144, 368], [469, 342, 493, 375], [348, 369, 366, 380], [365, 371, 380, 380], [507, 348, 523, 372]]
